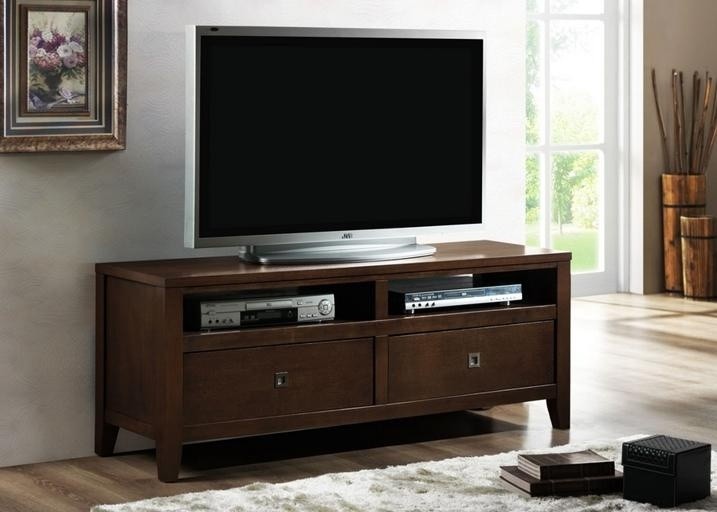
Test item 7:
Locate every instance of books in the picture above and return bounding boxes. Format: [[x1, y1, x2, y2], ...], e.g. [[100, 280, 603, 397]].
[[500, 465, 624, 497], [518, 450, 616, 479]]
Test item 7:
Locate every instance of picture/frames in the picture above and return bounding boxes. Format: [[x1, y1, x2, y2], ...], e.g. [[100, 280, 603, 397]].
[[0, 1, 126, 155]]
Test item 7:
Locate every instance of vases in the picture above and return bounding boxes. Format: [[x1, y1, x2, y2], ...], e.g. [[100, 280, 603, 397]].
[[661, 171, 716, 299]]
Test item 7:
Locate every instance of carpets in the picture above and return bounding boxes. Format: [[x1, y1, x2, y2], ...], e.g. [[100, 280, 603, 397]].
[[90, 433, 716, 511]]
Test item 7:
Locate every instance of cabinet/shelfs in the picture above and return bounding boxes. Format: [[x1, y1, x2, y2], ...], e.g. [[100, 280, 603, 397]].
[[94, 239, 571, 483]]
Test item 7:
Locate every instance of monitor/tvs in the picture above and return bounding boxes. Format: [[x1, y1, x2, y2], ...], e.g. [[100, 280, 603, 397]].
[[183, 24, 488, 265]]
